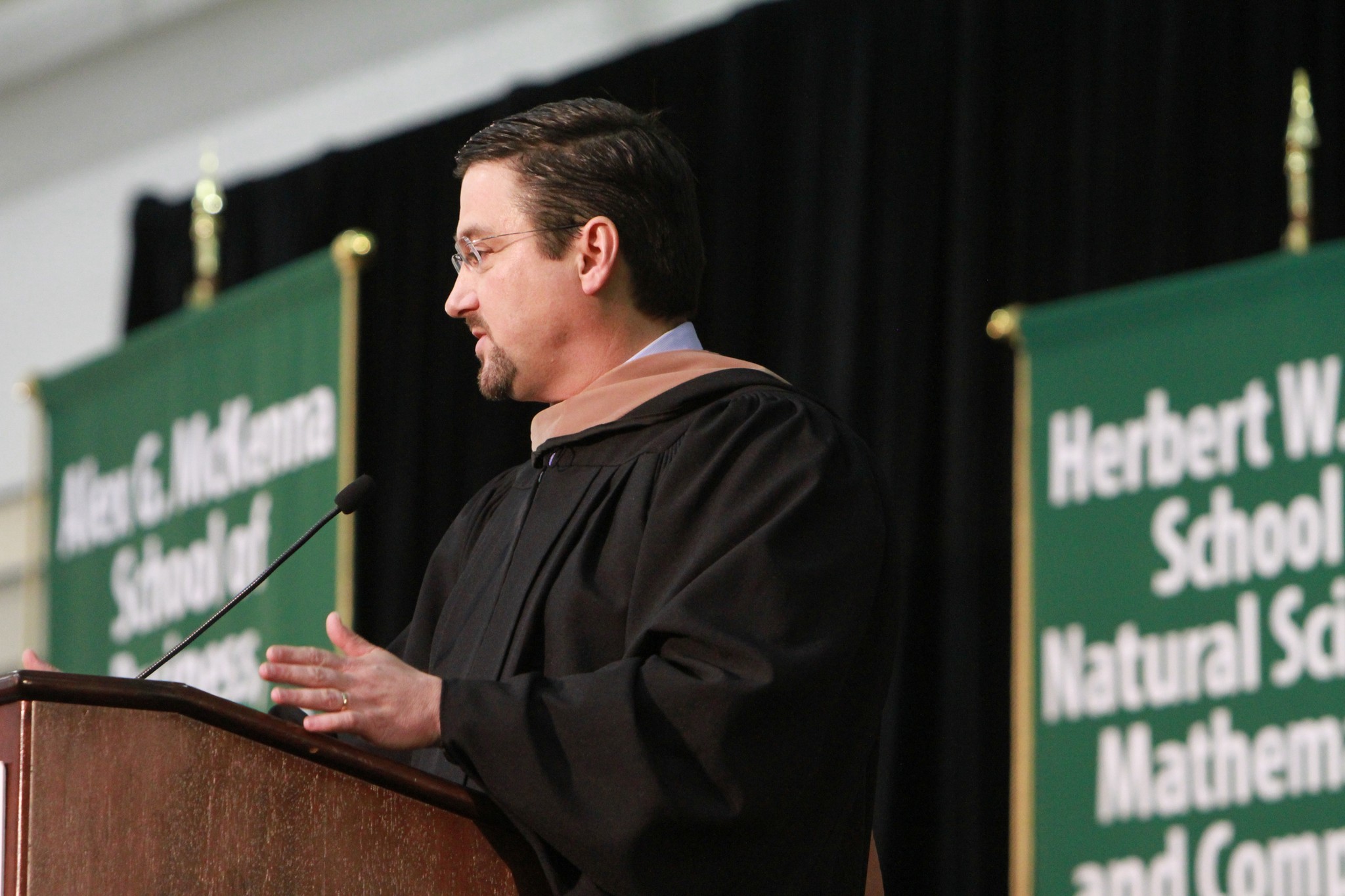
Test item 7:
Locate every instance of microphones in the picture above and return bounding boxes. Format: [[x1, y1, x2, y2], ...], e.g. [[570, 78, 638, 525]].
[[132, 475, 376, 679]]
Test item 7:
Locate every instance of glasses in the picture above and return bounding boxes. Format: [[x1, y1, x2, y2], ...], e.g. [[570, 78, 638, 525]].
[[451, 222, 587, 273]]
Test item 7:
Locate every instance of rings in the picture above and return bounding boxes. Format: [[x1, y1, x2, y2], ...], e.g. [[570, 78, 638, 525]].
[[340, 690, 347, 711]]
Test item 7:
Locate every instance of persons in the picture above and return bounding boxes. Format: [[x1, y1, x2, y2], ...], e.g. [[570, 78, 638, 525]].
[[21, 99, 897, 896]]
